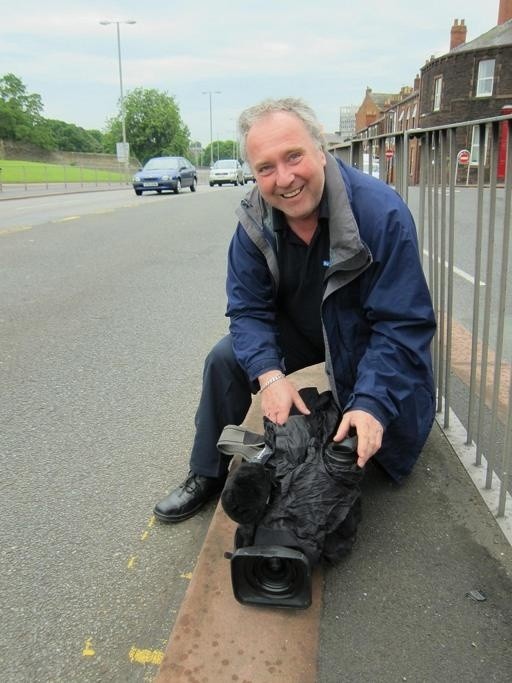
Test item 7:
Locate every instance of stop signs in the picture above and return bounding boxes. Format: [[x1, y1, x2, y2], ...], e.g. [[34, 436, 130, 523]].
[[457, 150, 470, 164], [384, 150, 393, 159]]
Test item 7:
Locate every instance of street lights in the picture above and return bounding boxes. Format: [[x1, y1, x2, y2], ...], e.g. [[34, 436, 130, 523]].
[[98, 20, 136, 168], [202, 90, 221, 166]]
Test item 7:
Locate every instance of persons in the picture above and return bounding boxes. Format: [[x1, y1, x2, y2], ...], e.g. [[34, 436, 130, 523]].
[[151, 95, 437, 524]]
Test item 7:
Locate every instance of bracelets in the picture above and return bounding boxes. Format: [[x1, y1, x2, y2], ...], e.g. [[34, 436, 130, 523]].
[[257, 371, 286, 398]]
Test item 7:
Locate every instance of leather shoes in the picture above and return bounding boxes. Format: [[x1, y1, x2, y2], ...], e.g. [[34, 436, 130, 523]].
[[153, 469, 229, 522]]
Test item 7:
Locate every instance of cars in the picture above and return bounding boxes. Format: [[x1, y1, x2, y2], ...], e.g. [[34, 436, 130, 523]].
[[132, 157, 198, 194], [208, 159, 256, 186]]
